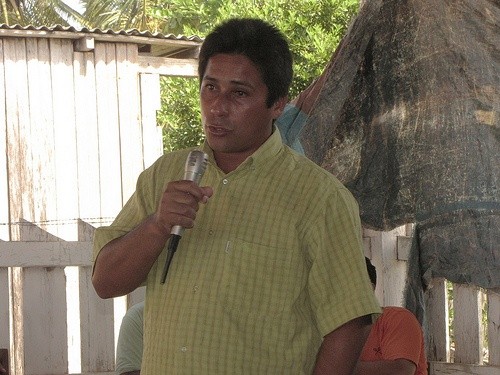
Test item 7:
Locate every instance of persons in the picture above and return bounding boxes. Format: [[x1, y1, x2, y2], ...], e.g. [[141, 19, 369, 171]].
[[356, 257, 430, 375], [89, 18, 383, 375]]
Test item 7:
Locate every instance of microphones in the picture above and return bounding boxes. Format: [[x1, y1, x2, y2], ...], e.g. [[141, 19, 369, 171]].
[[160, 151, 212, 284]]
